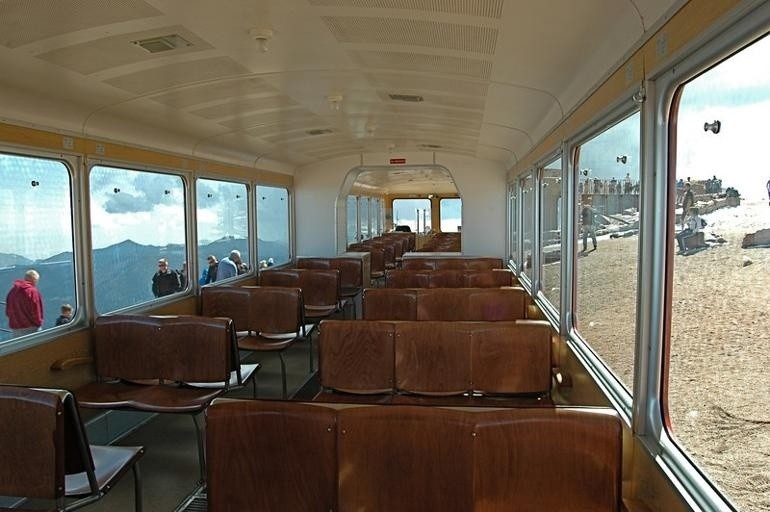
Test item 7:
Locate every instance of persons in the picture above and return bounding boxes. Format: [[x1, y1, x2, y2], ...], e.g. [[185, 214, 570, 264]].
[[152, 250, 274, 299], [55, 303, 73, 327], [713, 176, 717, 180], [578, 169, 702, 255], [5, 269, 45, 338], [766, 180, 770, 206]]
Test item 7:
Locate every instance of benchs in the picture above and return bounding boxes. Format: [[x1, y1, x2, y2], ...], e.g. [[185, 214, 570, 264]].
[[1, 384, 147, 512], [74, 314, 262, 483], [206, 397, 626, 511], [310, 320, 572, 408], [201, 285, 317, 400], [258, 258, 363, 320], [349, 232, 461, 287], [362, 286, 543, 322], [387, 258, 520, 286]]
